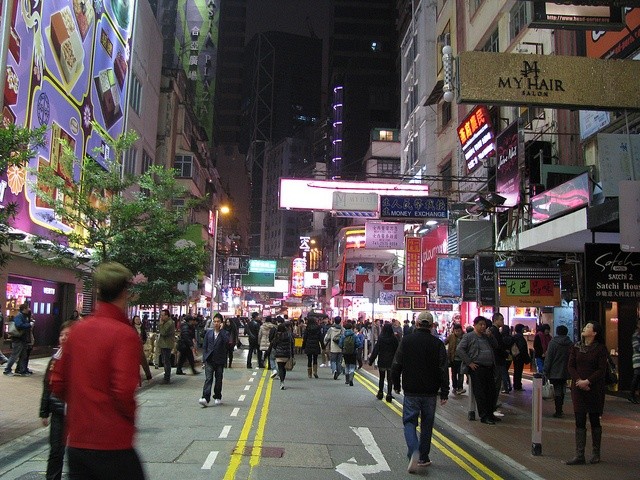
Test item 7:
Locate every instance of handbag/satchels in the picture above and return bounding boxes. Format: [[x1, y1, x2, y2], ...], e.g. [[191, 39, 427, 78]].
[[284, 339, 296, 370], [542, 380, 556, 400], [9, 321, 25, 337], [511, 338, 520, 357], [605, 354, 618, 385], [332, 330, 342, 344]]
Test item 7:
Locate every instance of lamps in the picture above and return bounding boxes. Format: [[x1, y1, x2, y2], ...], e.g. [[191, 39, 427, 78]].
[[486, 194, 506, 206], [474, 198, 491, 208]]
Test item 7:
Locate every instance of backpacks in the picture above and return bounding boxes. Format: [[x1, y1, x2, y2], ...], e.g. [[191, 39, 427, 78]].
[[343, 336, 354, 355]]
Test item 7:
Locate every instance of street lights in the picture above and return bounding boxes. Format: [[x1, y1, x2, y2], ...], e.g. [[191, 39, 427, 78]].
[[211, 204, 231, 315]]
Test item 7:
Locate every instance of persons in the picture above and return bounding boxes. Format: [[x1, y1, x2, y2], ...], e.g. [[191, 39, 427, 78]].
[[513, 323, 530, 391], [3, 304, 29, 377], [39, 320, 75, 480], [129, 309, 238, 406], [543, 326, 574, 417], [628, 319, 640, 404], [502, 322, 511, 396], [533, 324, 553, 373], [238, 311, 390, 403], [566, 320, 608, 465], [48, 262, 145, 479], [15, 305, 36, 374], [390, 310, 450, 472], [450, 312, 505, 426]]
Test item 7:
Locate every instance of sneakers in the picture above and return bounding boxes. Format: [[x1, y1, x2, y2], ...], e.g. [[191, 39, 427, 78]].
[[280, 383, 285, 390], [334, 370, 337, 380], [407, 450, 420, 472], [198, 398, 208, 406], [417, 458, 431, 465], [456, 388, 466, 395], [270, 370, 278, 378]]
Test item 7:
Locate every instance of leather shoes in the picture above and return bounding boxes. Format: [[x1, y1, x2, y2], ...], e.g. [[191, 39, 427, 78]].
[[481, 417, 495, 425], [489, 412, 501, 422]]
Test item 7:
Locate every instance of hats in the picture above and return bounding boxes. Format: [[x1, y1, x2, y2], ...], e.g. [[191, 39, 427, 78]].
[[417, 312, 433, 326]]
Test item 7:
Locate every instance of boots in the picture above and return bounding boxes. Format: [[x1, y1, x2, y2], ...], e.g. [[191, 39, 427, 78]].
[[308, 367, 312, 378], [349, 372, 354, 386], [313, 365, 319, 379], [345, 374, 349, 384], [589, 426, 602, 463], [566, 427, 587, 465]]
